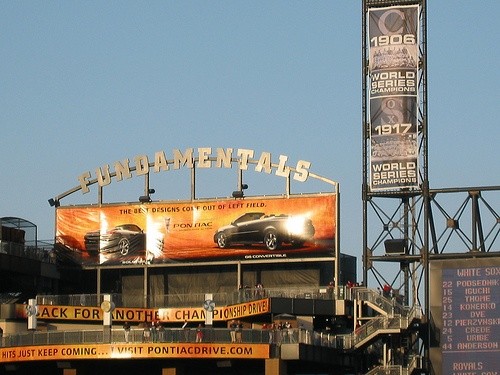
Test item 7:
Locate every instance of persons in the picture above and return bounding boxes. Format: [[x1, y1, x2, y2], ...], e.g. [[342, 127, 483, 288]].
[[375, 285, 391, 309], [195, 324, 204, 343], [367, 364, 376, 371], [254, 280, 265, 300], [123, 320, 130, 343], [277, 323, 283, 344], [356, 321, 363, 340], [346, 280, 363, 294], [235, 320, 243, 343], [268, 323, 275, 344], [262, 323, 267, 329], [285, 321, 296, 343], [329, 280, 335, 286], [150, 321, 165, 343], [229, 320, 236, 344]]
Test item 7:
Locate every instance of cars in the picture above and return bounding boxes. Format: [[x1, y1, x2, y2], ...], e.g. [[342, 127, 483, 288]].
[[213, 212, 315, 251], [84, 224, 164, 257]]
